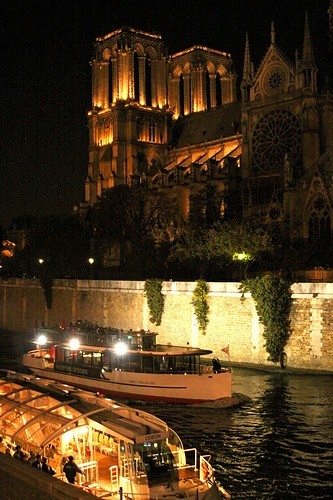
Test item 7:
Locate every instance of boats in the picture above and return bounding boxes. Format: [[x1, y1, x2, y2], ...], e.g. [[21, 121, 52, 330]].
[[0, 368, 232, 500], [20, 320, 233, 405]]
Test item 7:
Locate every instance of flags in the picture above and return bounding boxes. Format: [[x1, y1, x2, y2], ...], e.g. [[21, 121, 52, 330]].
[[221, 345, 229, 354]]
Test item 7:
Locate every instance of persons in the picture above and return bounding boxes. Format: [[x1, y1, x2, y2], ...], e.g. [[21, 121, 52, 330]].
[[0, 434, 89, 488], [211, 356, 222, 374], [32, 317, 135, 344]]
